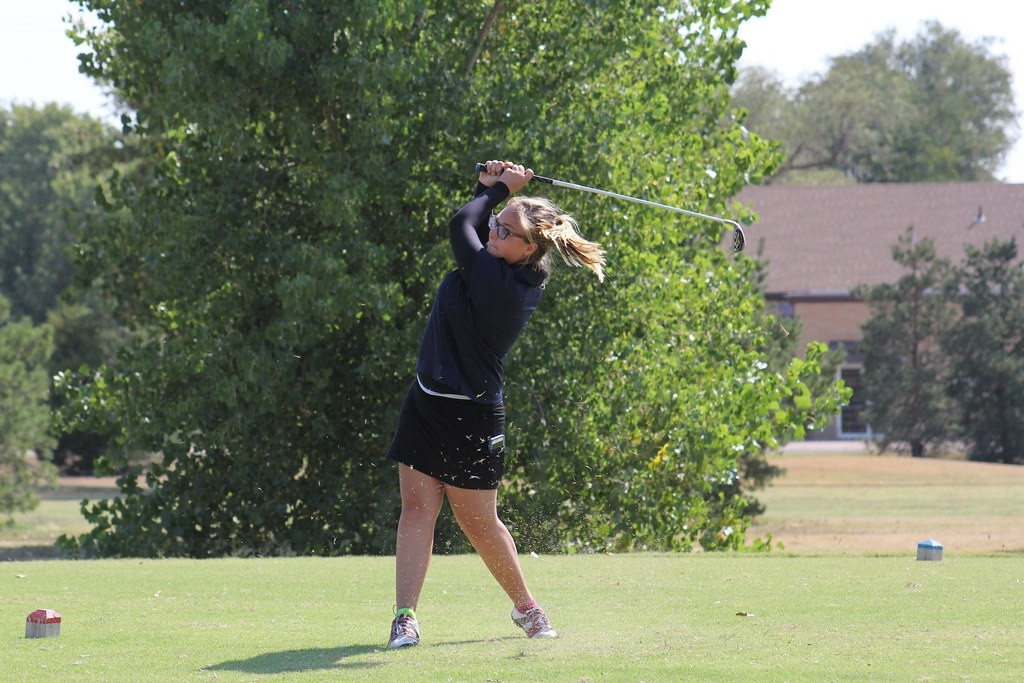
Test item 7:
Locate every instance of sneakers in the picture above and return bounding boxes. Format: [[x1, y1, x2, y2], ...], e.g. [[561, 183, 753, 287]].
[[511, 605, 558, 640], [387, 605, 420, 649]]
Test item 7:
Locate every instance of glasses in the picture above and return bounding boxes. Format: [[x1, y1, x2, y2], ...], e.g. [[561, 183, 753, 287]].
[[488, 214, 526, 240]]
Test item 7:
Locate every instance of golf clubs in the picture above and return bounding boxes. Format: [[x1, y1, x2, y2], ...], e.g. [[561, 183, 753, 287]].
[[475, 162, 745, 252]]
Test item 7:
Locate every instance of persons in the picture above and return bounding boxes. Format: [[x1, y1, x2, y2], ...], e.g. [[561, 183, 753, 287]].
[[388, 161, 605, 647]]
